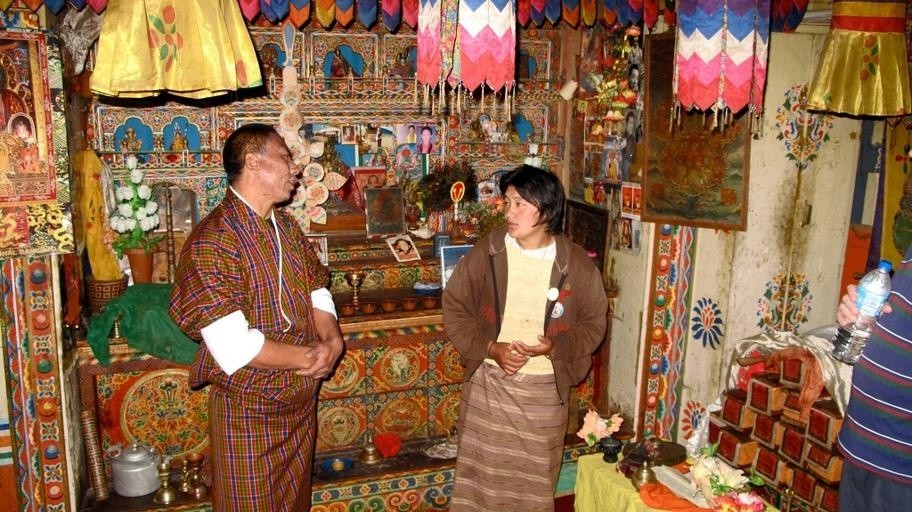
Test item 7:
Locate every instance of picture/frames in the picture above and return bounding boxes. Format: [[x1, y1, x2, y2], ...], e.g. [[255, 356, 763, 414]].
[[640, 32, 754, 231], [440, 245, 476, 289], [365, 187, 406, 239], [564, 198, 610, 286]]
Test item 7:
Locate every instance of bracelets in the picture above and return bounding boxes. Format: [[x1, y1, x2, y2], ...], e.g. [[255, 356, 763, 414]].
[[543, 351, 555, 360]]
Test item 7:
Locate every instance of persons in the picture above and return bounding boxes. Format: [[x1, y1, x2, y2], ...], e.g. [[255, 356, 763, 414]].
[[621, 188, 641, 212], [416, 126, 440, 155], [121, 127, 145, 153], [367, 174, 382, 188], [830, 241, 912, 511], [170, 130, 191, 151], [627, 62, 641, 93], [623, 112, 635, 152], [390, 238, 416, 261], [576, 103, 586, 122], [165, 120, 348, 512], [405, 124, 415, 143], [440, 163, 612, 511], [310, 240, 325, 264]]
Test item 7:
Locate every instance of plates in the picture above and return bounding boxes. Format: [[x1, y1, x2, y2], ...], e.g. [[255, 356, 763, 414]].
[[419, 442, 458, 460]]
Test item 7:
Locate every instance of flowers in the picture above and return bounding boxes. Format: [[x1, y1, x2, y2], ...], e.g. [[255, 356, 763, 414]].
[[105, 153, 165, 257], [701, 444, 767, 512], [576, 407, 623, 446]]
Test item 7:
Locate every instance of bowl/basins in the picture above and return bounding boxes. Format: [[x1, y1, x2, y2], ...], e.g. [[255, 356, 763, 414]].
[[321, 457, 352, 474]]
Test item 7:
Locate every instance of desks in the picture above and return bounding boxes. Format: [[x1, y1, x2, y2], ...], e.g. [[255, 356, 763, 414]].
[[573, 435, 784, 512]]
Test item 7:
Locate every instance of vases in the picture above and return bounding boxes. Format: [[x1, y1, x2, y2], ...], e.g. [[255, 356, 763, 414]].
[[124, 244, 158, 285]]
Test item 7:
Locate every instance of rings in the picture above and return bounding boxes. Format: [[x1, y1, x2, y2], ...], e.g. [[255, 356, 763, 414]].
[[528, 350, 533, 357]]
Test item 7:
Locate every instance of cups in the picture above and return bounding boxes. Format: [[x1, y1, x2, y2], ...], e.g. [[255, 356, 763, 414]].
[[420, 296, 439, 308], [359, 301, 378, 314], [400, 298, 419, 310], [337, 304, 358, 316], [379, 299, 400, 311]]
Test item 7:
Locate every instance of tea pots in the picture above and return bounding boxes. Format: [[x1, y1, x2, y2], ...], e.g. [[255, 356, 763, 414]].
[[104, 441, 174, 499]]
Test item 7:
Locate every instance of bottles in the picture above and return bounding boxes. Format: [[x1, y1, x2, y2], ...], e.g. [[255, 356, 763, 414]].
[[829, 258, 892, 365]]
